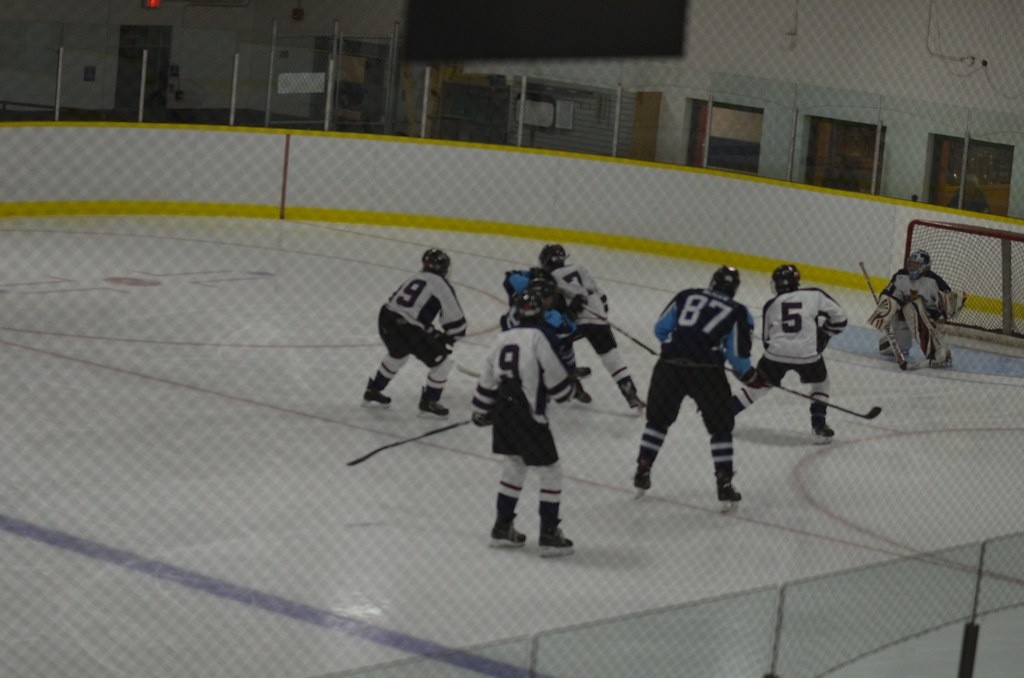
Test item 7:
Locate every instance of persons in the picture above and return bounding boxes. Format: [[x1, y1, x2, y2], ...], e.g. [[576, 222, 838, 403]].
[[726, 263, 848, 438], [632, 265, 767, 502], [361, 247, 468, 417], [868, 248, 967, 369], [499, 243, 647, 412], [471, 291, 581, 548]]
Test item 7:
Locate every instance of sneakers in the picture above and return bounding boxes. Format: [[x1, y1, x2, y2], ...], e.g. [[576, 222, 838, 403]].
[[628, 395, 646, 414], [539, 518, 574, 557], [714, 471, 741, 512], [416, 386, 449, 419], [812, 421, 835, 443], [927, 350, 953, 368], [490, 511, 525, 548], [361, 376, 391, 408], [634, 461, 650, 498]]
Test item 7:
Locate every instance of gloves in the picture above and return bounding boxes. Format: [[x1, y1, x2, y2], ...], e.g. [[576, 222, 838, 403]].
[[740, 366, 768, 388]]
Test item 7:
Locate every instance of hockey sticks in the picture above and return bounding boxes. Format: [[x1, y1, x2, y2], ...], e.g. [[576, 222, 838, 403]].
[[346, 415, 473, 469], [771, 380, 883, 421], [578, 303, 661, 358], [860, 261, 931, 370]]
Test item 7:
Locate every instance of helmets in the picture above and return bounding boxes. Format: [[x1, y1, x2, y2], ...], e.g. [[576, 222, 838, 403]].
[[540, 244, 565, 268], [771, 264, 801, 287], [422, 249, 450, 275], [529, 272, 556, 298], [904, 249, 930, 280], [510, 290, 542, 321], [709, 265, 739, 291]]
